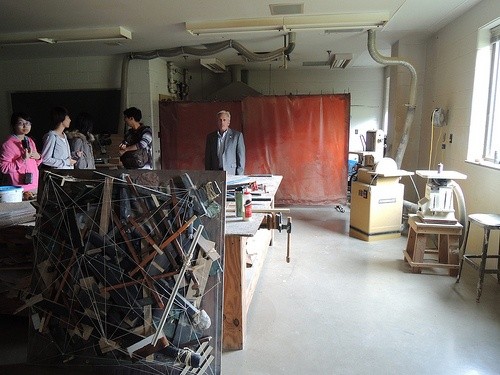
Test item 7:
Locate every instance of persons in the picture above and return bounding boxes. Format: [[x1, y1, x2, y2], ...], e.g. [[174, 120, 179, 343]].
[[41, 109, 76, 169], [0, 109, 41, 194], [117, 106, 154, 171], [204, 110, 246, 176], [66, 120, 96, 170]]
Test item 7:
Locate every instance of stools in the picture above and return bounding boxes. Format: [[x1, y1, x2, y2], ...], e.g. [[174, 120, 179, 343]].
[[456, 214, 500, 303]]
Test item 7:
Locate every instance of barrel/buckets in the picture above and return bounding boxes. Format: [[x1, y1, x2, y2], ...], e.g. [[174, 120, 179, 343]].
[[0, 186, 24, 204]]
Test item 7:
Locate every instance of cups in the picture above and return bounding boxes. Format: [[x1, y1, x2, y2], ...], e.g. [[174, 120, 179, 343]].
[[22, 140, 30, 150]]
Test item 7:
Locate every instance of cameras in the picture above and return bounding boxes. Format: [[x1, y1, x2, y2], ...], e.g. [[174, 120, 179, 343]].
[[22, 140, 29, 149]]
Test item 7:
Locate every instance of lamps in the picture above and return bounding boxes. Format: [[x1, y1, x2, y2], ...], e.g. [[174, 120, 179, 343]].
[[0, 27, 132, 48], [200, 58, 226, 73], [184, 10, 389, 36], [330, 54, 352, 70]]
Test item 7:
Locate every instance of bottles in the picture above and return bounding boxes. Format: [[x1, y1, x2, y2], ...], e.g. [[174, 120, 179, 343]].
[[235, 185, 248, 217], [243, 186, 252, 222]]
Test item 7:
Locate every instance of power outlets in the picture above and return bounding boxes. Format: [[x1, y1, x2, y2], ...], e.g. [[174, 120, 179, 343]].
[[450, 134, 452, 143]]
